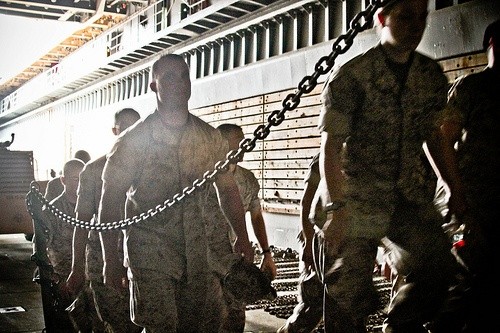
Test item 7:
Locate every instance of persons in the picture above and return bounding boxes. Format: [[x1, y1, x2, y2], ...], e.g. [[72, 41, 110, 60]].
[[217, 123, 276, 333], [43, 150, 91, 207], [25, 180, 45, 254], [99, 54, 254, 333], [32, 159, 107, 333], [66, 107, 144, 333], [277, 0, 500, 333]]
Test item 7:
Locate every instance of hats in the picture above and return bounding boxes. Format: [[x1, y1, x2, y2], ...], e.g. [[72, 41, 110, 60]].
[[89, 279, 144, 333], [64, 286, 109, 333], [220, 257, 278, 307]]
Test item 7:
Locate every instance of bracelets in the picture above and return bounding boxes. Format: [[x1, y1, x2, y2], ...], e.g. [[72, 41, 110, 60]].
[[262, 249, 270, 253]]
